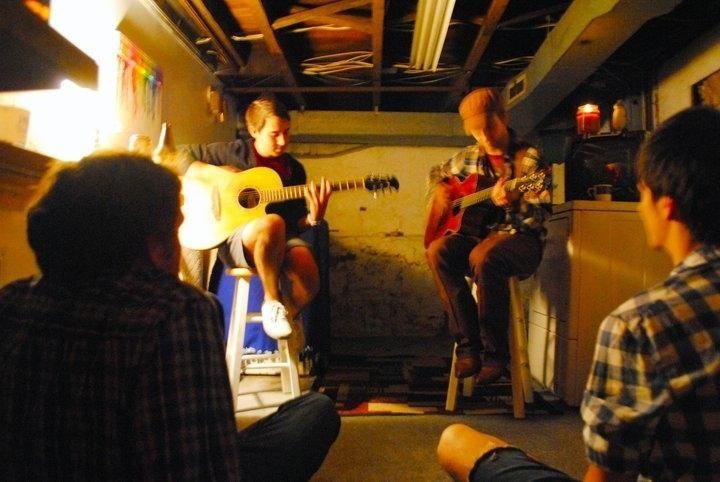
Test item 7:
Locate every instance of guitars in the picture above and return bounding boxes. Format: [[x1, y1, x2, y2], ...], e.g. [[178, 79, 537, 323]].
[[424, 171, 552, 249], [178, 162, 399, 251]]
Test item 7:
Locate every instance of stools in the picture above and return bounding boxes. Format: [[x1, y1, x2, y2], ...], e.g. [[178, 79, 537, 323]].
[[444, 267, 537, 420], [222, 267, 300, 410]]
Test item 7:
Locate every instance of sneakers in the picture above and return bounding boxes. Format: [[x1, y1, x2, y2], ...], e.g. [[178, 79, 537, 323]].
[[289, 321, 306, 352], [454, 355, 510, 385], [261, 301, 293, 340]]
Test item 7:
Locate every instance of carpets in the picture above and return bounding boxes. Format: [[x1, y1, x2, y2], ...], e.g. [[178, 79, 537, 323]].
[[307, 354, 568, 417]]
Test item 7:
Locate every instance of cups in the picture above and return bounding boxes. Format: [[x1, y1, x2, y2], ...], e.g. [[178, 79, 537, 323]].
[[587, 184, 613, 201]]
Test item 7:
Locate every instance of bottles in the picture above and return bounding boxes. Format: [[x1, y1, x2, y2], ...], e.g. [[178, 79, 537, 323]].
[[576, 106, 601, 135]]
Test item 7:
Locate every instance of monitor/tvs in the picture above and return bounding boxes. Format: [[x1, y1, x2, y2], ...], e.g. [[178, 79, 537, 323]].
[[565, 129, 651, 201]]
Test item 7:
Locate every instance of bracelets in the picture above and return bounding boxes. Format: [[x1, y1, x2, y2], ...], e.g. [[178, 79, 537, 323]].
[[306, 214, 322, 228]]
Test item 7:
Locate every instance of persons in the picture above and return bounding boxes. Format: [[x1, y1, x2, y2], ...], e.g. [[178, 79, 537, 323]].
[[436, 106, 719, 480], [172, 97, 334, 340], [1, 151, 341, 479], [426, 88, 555, 385]]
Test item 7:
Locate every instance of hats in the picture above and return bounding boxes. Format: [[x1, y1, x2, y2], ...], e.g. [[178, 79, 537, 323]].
[[458, 87, 504, 131]]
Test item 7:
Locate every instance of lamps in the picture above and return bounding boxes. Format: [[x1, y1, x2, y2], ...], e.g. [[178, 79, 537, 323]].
[[575, 102, 601, 138]]
